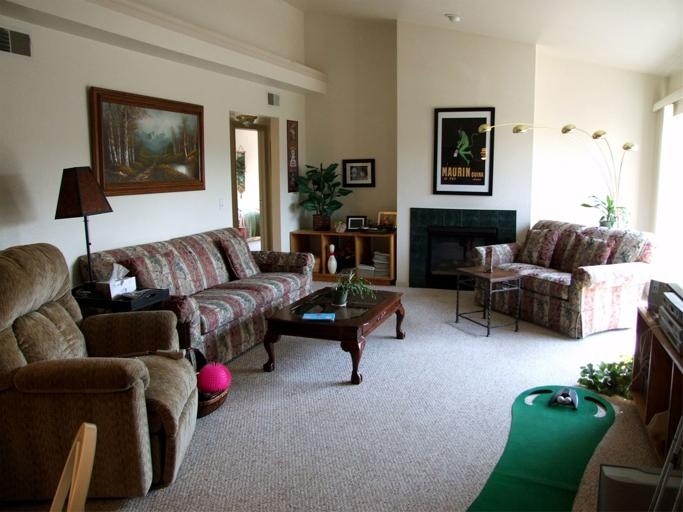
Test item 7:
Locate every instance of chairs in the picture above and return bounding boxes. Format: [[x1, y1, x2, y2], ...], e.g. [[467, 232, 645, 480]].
[[49, 421, 98, 511]]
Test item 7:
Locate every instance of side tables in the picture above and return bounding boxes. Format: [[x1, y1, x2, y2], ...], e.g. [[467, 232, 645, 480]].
[[86, 295, 188, 319]]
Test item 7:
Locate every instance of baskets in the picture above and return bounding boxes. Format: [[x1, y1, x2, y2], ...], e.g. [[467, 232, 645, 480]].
[[197, 388, 229, 418]]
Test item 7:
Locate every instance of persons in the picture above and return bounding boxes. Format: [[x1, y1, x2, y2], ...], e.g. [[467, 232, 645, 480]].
[[454, 127, 474, 166]]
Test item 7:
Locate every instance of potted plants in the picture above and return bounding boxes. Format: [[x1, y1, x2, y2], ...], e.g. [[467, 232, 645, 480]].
[[296, 162, 353, 232], [581, 195, 617, 227], [327, 268, 377, 305]]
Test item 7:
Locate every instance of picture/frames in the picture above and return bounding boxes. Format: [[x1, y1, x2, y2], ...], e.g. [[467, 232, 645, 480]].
[[347, 216, 367, 231], [433, 107, 496, 195], [377, 212, 397, 226], [87, 88, 206, 196], [341, 158, 375, 186]]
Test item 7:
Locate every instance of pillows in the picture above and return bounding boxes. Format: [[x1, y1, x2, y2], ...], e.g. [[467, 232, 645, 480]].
[[517, 229, 561, 267], [559, 231, 615, 271], [125, 250, 181, 297], [219, 236, 260, 277]]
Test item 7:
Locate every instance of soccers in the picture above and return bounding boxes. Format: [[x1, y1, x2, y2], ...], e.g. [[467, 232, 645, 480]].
[[196, 362, 230, 391]]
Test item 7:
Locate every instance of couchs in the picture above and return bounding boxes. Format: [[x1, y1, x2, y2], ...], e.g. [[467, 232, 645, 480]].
[[0, 243, 201, 499], [79, 230, 314, 366], [471, 218, 659, 338]]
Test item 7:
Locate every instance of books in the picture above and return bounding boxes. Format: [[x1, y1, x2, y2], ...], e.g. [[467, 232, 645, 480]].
[[372, 250, 390, 278]]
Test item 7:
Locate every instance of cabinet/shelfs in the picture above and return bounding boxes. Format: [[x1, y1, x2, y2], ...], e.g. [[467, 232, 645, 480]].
[[290, 231, 395, 285], [632, 306, 683, 473]]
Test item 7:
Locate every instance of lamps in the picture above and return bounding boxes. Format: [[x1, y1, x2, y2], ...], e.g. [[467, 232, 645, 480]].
[[55, 167, 113, 280], [478, 120, 639, 207]]
